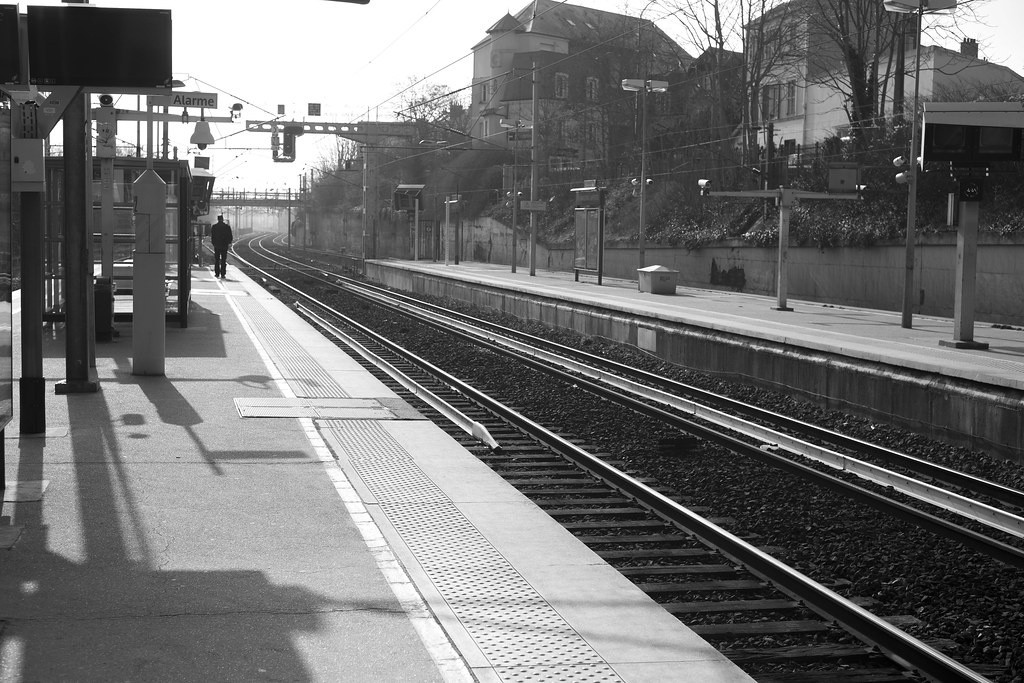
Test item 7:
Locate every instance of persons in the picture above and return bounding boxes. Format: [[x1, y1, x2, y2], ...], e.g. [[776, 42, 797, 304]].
[[211, 214, 234, 278]]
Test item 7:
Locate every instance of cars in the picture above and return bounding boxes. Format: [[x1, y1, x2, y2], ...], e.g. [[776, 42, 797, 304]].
[[788, 154, 813, 178]]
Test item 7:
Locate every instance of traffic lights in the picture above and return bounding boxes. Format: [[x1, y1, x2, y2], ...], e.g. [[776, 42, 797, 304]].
[[284, 124, 304, 156]]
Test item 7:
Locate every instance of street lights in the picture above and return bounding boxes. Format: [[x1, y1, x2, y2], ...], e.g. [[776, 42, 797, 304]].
[[883, 0, 957, 328], [622, 78, 669, 292], [419, 139, 448, 262], [499, 118, 533, 273]]
